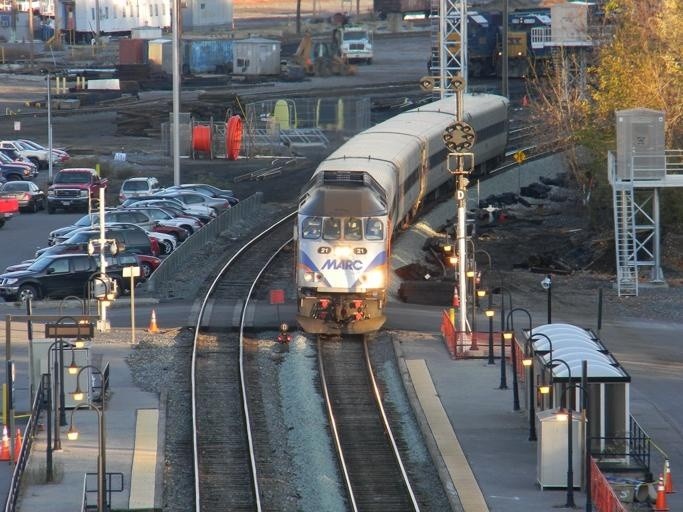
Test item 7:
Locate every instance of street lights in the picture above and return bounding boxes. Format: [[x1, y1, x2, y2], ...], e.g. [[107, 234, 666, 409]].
[[46, 271, 115, 512]]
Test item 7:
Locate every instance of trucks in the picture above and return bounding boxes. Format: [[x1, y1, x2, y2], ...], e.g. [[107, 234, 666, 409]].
[[335, 26, 375, 65]]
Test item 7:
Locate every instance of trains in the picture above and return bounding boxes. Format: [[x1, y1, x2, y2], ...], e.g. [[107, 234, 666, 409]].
[[292, 91, 511, 335], [427, 9, 552, 78]]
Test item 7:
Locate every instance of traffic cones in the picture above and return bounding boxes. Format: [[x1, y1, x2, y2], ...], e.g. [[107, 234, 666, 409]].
[[0, 424, 23, 465], [148, 308, 161, 333], [655, 473, 670, 512], [663, 459, 675, 493]]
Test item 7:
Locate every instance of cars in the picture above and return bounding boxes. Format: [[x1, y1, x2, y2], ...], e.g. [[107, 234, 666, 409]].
[[0, 139, 238, 302]]
